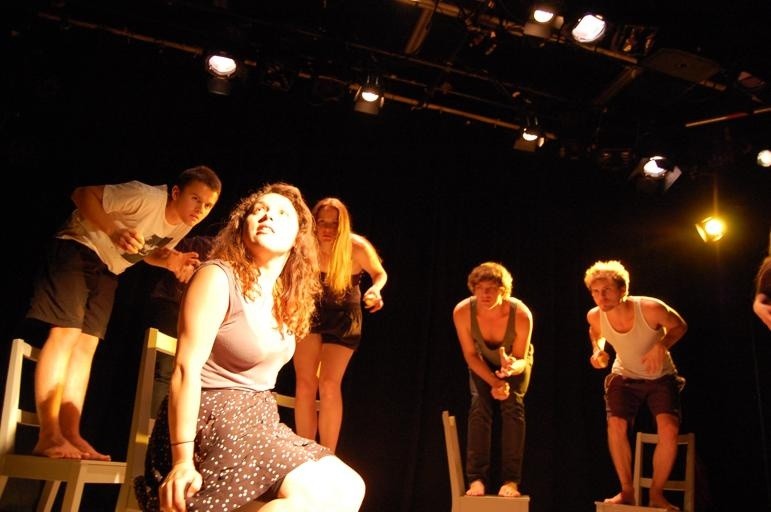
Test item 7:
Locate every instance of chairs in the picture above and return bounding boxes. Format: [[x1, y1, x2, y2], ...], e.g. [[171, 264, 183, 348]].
[[0, 339, 126, 512], [271, 391, 320, 411], [442, 411, 530, 512], [593, 433, 695, 512], [115, 328, 177, 512]]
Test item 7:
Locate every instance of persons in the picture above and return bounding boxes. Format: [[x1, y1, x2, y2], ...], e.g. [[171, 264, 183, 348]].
[[26, 165, 223, 460], [451, 260, 534, 497], [294, 196, 390, 453], [583, 260, 688, 512], [754, 238, 771, 331], [131, 182, 368, 512]]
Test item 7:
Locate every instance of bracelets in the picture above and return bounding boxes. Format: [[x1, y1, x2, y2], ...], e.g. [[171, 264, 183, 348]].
[[169, 439, 196, 446]]
